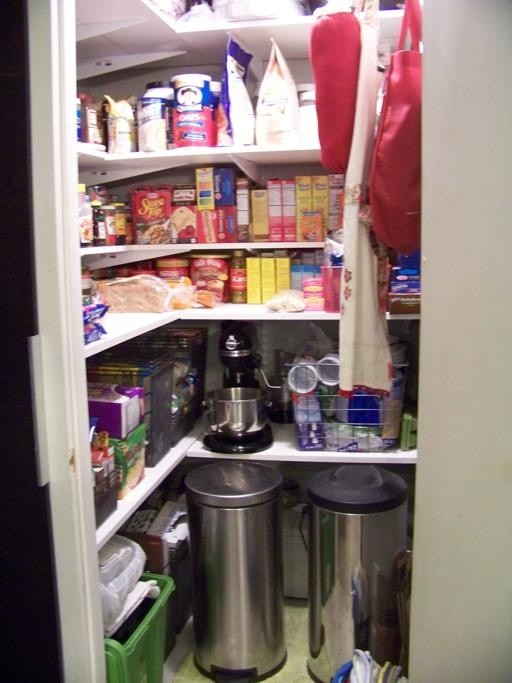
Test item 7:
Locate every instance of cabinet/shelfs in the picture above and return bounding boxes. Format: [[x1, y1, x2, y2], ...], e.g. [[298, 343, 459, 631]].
[[73, 0, 420, 552]]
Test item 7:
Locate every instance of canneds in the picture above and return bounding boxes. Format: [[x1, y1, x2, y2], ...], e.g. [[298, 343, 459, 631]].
[[91, 202, 126, 246]]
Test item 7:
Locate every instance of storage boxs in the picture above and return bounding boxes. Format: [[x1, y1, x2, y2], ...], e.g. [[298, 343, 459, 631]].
[[96, 495, 191, 682]]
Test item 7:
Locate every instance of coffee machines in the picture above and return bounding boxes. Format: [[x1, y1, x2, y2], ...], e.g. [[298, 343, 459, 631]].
[[265, 350, 298, 425]]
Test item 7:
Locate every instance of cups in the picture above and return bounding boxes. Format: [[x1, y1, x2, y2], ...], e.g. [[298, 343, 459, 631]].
[[287, 363, 318, 393], [316, 354, 340, 387]]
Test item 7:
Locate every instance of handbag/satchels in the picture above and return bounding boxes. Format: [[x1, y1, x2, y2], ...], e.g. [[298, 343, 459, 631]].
[[368, 0, 423, 258]]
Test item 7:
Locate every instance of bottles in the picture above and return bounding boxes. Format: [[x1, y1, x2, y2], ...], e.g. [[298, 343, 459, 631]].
[[229, 248, 246, 304], [295, 84, 323, 149], [78, 186, 94, 245]]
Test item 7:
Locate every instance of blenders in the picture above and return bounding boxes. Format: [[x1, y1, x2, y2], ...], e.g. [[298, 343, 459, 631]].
[[200, 325, 274, 454]]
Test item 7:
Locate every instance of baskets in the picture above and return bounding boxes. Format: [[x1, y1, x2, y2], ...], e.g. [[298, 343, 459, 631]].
[[284, 359, 409, 452]]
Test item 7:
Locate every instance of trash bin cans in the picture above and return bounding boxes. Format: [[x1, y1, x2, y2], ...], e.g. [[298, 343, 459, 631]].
[[184, 460, 288, 683], [298, 465, 410, 683]]
